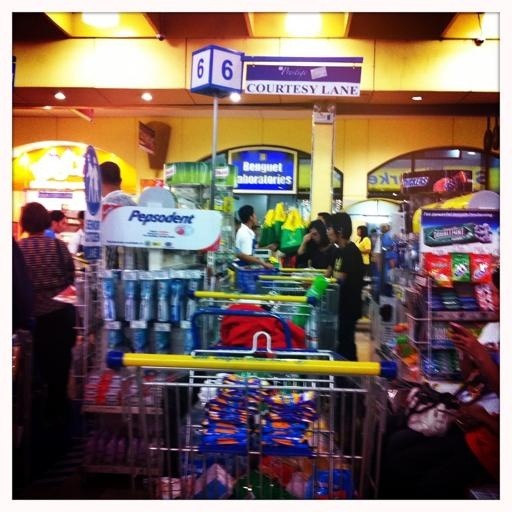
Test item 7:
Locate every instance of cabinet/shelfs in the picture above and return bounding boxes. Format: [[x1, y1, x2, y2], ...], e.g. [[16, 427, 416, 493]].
[[406, 269, 500, 381]]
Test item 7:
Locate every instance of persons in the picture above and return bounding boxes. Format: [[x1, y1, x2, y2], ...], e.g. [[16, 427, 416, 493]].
[[12, 201, 87, 462], [252, 224, 277, 251], [363, 266, 500, 500], [378, 393, 499, 499], [99, 162, 138, 209], [235, 204, 277, 270], [297, 210, 396, 363]]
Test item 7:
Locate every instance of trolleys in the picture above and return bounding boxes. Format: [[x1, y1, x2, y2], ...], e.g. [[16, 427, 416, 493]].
[[104, 268, 399, 500]]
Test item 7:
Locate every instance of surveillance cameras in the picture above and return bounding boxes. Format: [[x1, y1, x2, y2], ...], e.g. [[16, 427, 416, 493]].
[[156, 33, 166, 41], [476, 38, 485, 43]]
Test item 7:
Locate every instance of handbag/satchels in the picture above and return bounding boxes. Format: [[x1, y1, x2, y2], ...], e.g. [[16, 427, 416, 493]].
[[405, 387, 447, 435]]
